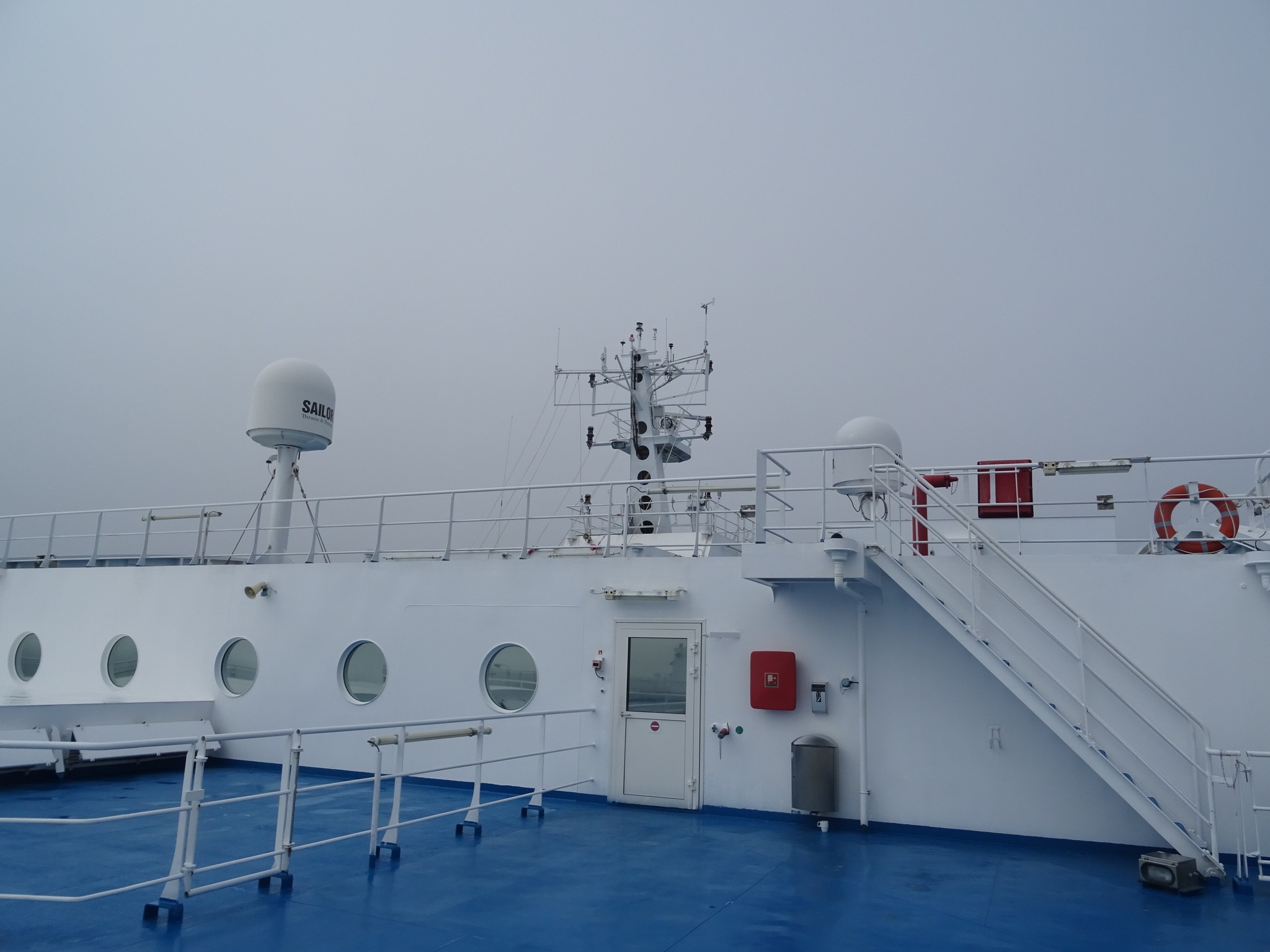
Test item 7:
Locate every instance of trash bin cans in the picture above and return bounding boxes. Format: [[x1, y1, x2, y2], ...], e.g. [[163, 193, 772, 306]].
[[789, 733, 838, 815]]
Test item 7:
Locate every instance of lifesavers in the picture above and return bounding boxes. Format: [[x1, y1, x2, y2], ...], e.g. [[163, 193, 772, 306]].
[[1154, 482, 1240, 556]]
